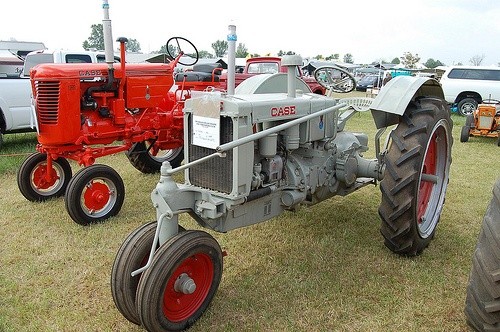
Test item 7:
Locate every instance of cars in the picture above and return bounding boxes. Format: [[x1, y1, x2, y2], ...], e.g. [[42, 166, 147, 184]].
[[357, 75, 383, 92]]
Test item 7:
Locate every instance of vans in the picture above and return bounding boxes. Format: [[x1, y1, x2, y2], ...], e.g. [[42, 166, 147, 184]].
[[439, 65, 500, 118]]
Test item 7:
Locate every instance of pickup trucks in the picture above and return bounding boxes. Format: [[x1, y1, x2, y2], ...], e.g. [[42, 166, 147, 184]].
[[217, 56, 326, 95], [0, 49, 147, 146]]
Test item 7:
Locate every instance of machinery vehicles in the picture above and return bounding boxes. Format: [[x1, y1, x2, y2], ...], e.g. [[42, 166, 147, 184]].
[[110, 25, 455, 332], [16, 1, 240, 227], [459, 99, 500, 148]]
